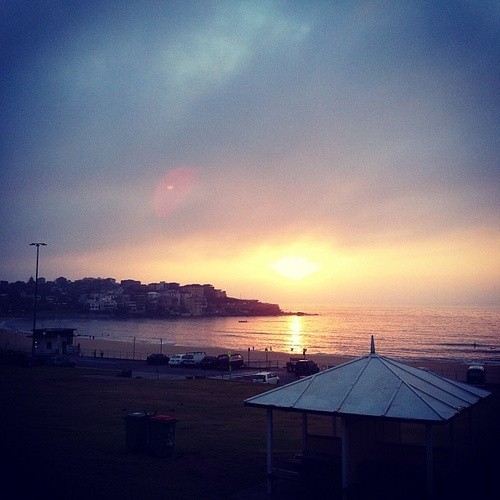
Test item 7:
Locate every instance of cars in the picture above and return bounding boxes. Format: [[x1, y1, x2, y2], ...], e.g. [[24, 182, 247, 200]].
[[147, 354, 168, 365]]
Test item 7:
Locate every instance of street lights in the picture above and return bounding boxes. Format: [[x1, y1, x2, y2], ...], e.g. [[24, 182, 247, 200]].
[[29, 243, 48, 354]]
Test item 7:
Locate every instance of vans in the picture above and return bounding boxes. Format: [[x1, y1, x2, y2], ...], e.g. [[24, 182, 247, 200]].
[[296, 359, 319, 376]]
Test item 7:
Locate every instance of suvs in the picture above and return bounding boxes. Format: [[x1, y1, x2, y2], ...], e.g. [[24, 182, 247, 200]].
[[215, 353, 244, 371], [466, 366, 483, 381], [252, 372, 280, 385], [169, 353, 215, 370]]
